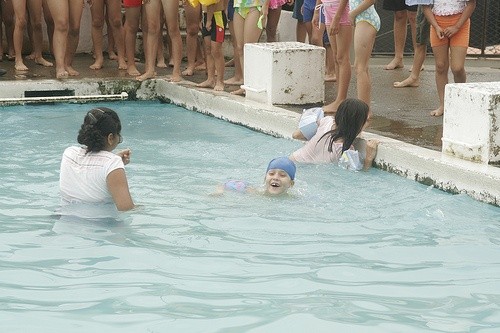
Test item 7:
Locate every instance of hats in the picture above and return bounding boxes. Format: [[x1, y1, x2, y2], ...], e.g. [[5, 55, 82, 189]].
[[267, 157, 296, 180]]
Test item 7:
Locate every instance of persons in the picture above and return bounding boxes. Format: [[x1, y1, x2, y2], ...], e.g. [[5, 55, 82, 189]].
[[286, 97, 382, 173], [423, 0, 475, 117], [348, 0, 381, 128], [209, 156, 297, 203], [59, 107, 135, 212], [0, 0, 337, 95], [382, 0, 425, 72], [393, 0, 434, 88], [312, 0, 352, 112]]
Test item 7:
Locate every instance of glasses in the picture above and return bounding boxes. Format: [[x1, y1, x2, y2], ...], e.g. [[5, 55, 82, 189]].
[[106, 131, 123, 143]]
